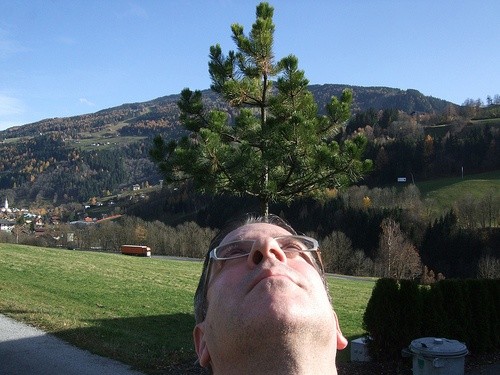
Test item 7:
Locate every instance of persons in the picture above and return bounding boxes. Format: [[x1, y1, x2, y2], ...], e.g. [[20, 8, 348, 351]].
[[193, 211, 348, 375]]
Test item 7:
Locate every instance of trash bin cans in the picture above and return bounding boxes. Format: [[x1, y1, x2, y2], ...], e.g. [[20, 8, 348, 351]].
[[408, 336, 469, 375]]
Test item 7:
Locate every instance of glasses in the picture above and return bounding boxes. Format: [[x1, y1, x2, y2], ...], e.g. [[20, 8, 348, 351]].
[[202, 235, 326, 320]]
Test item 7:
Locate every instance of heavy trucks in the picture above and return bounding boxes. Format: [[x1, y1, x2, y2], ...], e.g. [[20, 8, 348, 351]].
[[121, 245, 150, 257]]
